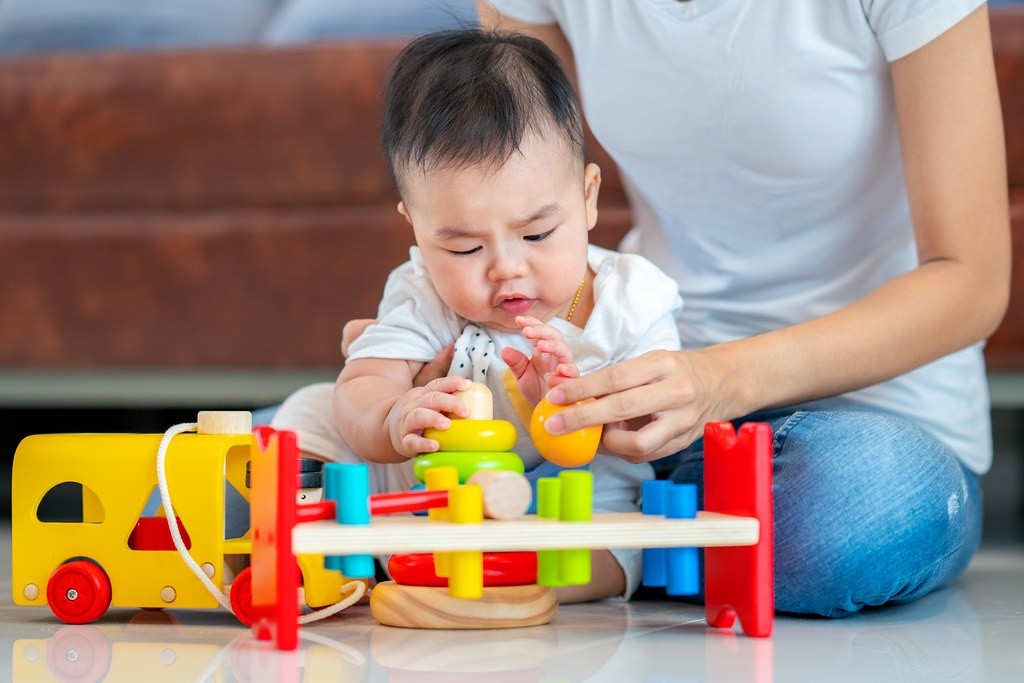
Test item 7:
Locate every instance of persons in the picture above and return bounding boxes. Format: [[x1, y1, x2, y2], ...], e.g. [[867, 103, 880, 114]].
[[143, 0, 1013, 616], [270, 32, 687, 607]]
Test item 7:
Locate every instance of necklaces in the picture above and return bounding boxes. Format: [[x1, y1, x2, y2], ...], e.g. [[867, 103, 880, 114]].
[[566, 269, 587, 321]]
